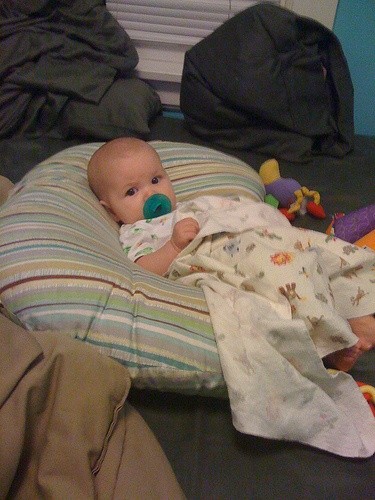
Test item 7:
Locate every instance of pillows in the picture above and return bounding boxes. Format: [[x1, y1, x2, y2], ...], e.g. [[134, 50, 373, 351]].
[[42, 77, 164, 140], [0, 139, 267, 399]]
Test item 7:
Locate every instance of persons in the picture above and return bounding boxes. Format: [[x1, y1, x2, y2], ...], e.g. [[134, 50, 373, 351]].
[[87, 137, 375, 373]]
